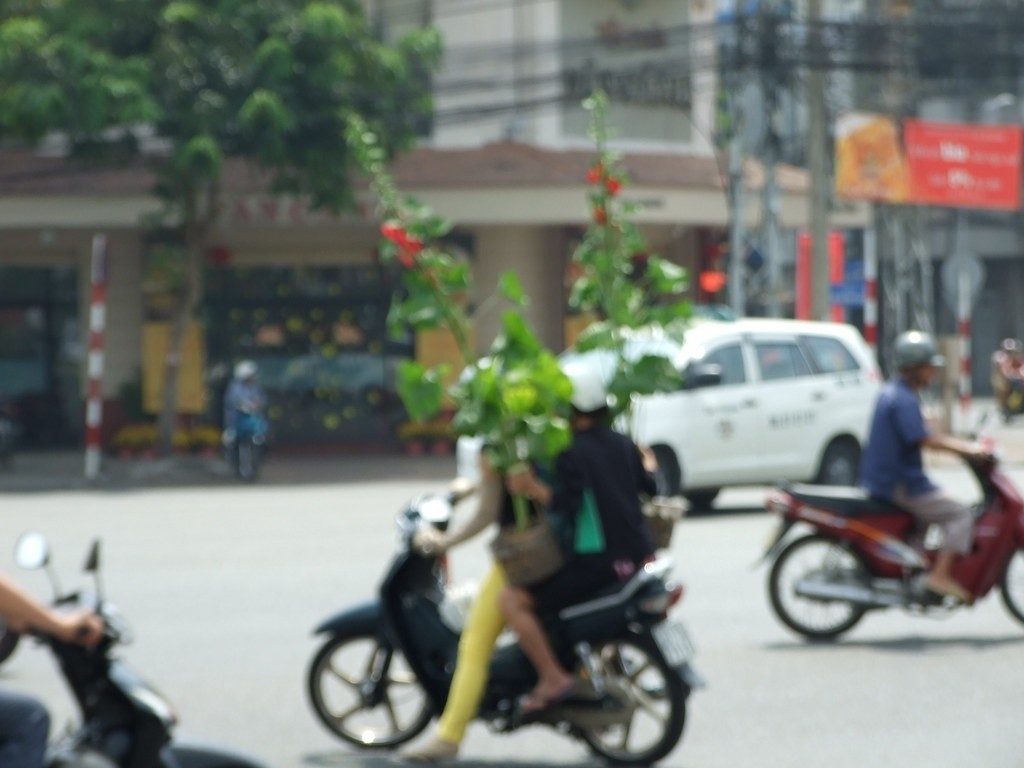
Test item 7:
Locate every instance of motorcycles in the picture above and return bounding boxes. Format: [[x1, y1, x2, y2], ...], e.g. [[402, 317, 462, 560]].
[[302, 473, 703, 767], [733, 409, 1024, 630]]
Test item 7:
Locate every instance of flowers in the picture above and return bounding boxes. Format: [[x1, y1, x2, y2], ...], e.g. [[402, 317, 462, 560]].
[[348, 112, 568, 527], [569, 93, 702, 437]]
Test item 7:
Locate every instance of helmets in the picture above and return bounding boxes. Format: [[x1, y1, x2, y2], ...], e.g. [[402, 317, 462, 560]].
[[890, 329, 947, 369], [554, 351, 619, 412]]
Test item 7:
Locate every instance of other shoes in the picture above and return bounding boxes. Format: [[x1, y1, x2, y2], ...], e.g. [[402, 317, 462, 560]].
[[923, 572, 973, 604], [390, 716, 461, 767]]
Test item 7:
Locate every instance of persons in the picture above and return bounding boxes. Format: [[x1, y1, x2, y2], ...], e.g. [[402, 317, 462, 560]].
[[384, 350, 650, 768], [989, 335, 1024, 422], [0, 574, 107, 768], [860, 328, 990, 609], [221, 358, 276, 470]]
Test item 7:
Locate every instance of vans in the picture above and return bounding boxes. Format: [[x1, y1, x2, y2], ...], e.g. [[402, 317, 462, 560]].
[[454, 309, 893, 514]]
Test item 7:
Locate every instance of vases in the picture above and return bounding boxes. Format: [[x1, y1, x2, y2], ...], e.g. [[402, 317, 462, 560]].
[[640, 512, 675, 547], [487, 524, 563, 591]]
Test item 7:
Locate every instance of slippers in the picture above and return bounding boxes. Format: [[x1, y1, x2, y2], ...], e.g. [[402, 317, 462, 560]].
[[509, 679, 575, 724]]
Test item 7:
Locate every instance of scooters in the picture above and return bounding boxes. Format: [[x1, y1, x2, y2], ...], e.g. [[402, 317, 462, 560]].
[[0, 532, 273, 767]]
